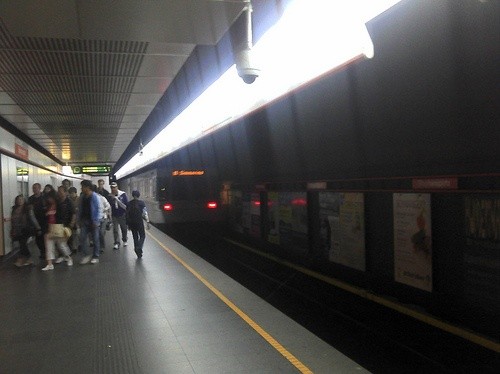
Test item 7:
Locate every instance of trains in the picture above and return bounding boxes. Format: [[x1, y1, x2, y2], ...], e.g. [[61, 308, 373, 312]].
[[119, 168, 222, 241]]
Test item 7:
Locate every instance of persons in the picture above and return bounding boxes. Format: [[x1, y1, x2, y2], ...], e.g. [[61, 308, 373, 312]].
[[11, 179, 127, 271], [125, 191, 150, 258]]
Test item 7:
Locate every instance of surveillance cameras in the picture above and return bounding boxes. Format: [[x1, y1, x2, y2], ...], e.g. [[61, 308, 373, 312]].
[[235, 50, 261, 84]]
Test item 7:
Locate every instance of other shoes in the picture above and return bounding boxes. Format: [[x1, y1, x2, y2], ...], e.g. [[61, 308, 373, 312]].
[[113, 244, 119, 249], [123, 241, 127, 246], [41, 265, 54, 270], [90, 258, 100, 263], [15, 258, 23, 268], [67, 257, 74, 266], [79, 255, 92, 263], [56, 257, 66, 263]]
